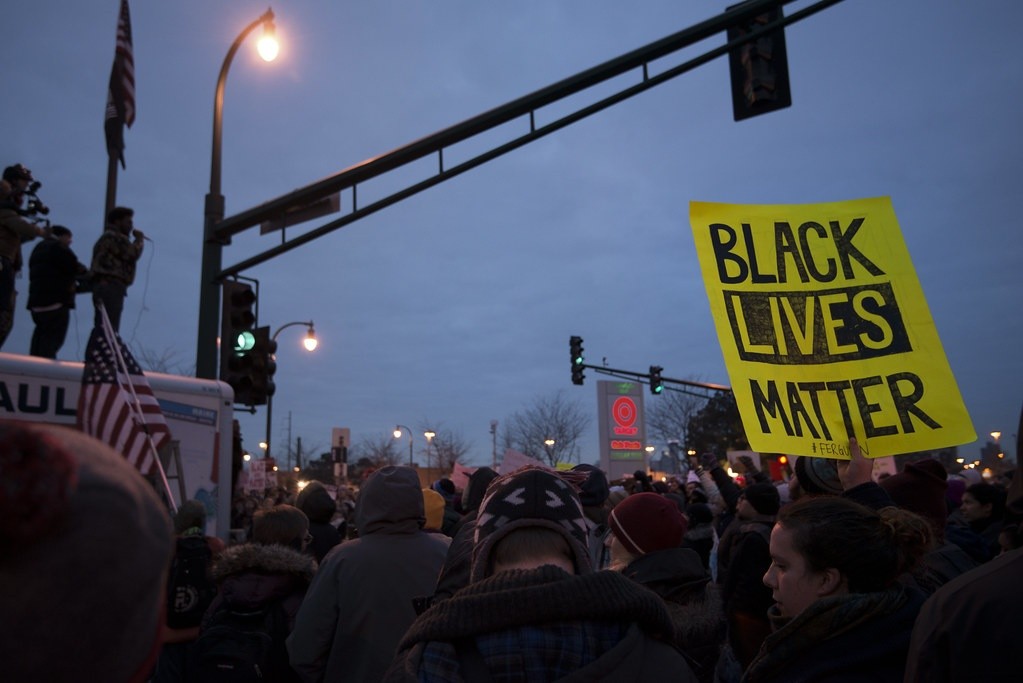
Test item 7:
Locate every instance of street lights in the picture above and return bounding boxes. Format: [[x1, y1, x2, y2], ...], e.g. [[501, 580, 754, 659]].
[[195, 7, 279, 380], [394, 425, 412, 466], [265, 320, 318, 458]]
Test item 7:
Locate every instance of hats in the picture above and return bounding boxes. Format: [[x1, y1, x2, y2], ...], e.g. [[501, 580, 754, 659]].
[[1, 419, 177, 683], [609, 491, 689, 557], [173, 499, 207, 535], [745, 482, 780, 514], [794, 455, 843, 496], [254, 504, 310, 546], [470, 465, 591, 583], [880, 459, 947, 544], [431, 478, 455, 502]]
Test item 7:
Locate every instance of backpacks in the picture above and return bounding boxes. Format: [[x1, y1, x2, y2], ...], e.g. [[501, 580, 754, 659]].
[[192, 576, 311, 683]]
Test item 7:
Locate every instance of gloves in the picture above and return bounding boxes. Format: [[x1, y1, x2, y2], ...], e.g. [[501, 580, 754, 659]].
[[703, 453, 717, 469]]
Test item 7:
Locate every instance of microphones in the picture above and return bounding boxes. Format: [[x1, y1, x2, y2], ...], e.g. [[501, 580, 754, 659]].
[[143, 235, 150, 241]]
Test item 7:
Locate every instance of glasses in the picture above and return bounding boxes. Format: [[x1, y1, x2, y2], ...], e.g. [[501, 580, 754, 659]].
[[302, 534, 312, 544]]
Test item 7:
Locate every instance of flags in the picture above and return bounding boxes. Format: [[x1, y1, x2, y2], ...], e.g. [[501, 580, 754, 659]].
[[79, 322, 170, 477], [103, 0, 136, 168]]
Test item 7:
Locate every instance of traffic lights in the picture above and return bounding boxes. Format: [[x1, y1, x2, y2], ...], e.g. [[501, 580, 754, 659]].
[[570, 336, 586, 386], [241, 325, 278, 406], [650, 365, 664, 395], [220, 279, 258, 375]]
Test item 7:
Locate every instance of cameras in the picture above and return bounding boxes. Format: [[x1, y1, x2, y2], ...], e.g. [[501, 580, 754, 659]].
[[27, 199, 49, 215]]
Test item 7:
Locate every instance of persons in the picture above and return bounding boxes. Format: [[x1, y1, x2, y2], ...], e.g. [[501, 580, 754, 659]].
[[0, 163, 145, 361], [1, 425, 1023, 683]]
